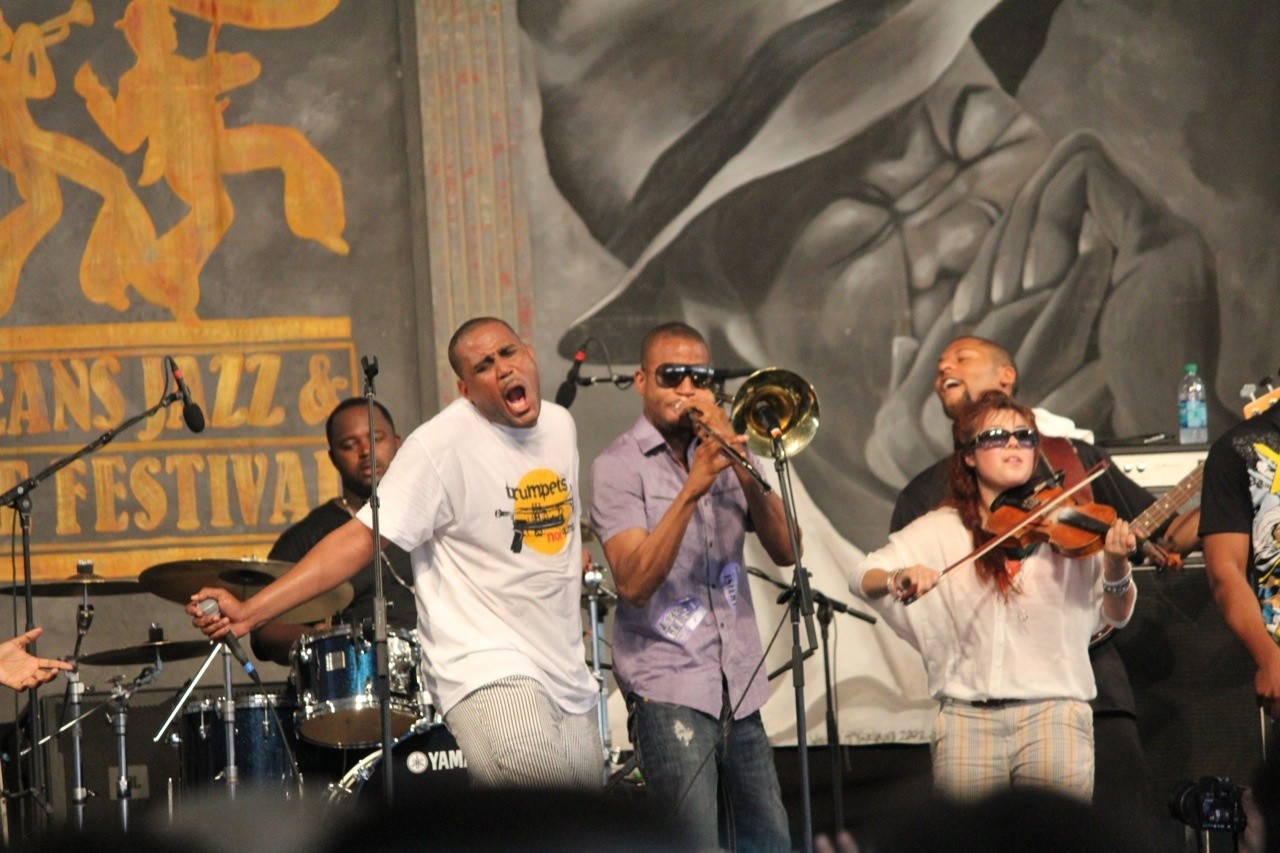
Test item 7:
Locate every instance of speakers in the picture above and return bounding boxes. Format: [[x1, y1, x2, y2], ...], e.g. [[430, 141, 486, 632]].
[[1120, 559, 1273, 853], [20, 688, 192, 833]]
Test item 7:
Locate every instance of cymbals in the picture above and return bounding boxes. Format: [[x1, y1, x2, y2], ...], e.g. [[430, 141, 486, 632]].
[[0, 573, 149, 598], [138, 557, 356, 625], [76, 639, 216, 666]]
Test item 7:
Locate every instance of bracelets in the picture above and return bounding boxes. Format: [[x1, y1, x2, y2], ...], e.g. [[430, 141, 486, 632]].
[[888, 569, 901, 598], [1102, 561, 1132, 595]]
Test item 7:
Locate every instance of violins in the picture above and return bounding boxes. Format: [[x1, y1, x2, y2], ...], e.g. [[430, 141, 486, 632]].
[[981, 484, 1184, 574]]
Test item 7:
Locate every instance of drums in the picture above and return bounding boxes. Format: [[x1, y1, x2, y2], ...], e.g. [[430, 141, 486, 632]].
[[318, 721, 459, 837], [171, 689, 301, 825], [290, 622, 424, 751]]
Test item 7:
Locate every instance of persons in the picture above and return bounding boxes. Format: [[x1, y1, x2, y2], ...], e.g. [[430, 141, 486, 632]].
[[249, 397, 419, 664], [854, 391, 1136, 802], [0, 627, 72, 692], [891, 337, 1199, 846], [187, 318, 604, 783], [587, 324, 804, 853], [1200, 399, 1280, 853]]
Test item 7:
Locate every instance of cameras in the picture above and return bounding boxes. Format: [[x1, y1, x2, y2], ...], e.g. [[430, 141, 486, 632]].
[[1167, 775, 1247, 833]]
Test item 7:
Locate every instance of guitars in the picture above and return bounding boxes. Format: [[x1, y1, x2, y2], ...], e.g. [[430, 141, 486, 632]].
[[1120, 461, 1203, 544]]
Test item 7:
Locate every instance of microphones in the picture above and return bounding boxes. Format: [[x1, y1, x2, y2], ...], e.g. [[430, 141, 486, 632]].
[[555, 340, 588, 409], [755, 401, 781, 440], [168, 359, 205, 434], [198, 599, 262, 686]]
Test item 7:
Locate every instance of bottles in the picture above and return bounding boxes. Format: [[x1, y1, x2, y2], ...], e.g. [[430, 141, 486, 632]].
[[1177, 363, 1208, 445]]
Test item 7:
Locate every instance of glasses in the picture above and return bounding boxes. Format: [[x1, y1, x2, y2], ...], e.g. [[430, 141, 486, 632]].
[[652, 362, 721, 391], [964, 427, 1046, 452]]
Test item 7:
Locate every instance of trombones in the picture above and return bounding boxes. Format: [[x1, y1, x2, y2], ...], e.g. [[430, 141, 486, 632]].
[[674, 366, 821, 493]]
